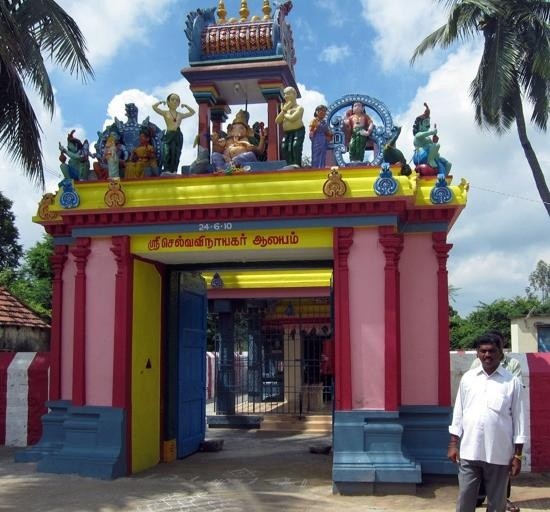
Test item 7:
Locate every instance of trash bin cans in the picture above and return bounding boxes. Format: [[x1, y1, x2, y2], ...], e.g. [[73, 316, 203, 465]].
[[303, 387, 323, 411], [162, 439, 177, 463]]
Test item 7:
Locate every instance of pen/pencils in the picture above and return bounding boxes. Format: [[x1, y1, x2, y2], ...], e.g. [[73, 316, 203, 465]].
[[456, 460, 460, 463]]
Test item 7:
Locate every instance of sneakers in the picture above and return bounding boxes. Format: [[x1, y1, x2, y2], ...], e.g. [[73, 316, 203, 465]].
[[506, 499, 520, 512]]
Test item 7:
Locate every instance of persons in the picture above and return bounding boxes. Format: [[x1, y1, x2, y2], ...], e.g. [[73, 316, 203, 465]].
[[272, 86, 306, 169], [153, 92, 195, 173], [77, 140, 95, 181], [411, 100, 452, 178], [343, 101, 376, 162], [58, 139, 82, 182], [308, 105, 334, 169], [124, 128, 158, 180], [103, 140, 126, 182], [92, 131, 130, 180], [468, 326, 523, 512], [445, 336, 527, 512]]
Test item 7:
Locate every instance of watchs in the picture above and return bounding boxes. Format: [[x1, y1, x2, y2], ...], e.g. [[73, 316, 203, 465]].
[[513, 453, 523, 460]]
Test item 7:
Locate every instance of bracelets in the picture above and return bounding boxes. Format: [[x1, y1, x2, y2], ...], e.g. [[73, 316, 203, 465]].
[[446, 443, 459, 448]]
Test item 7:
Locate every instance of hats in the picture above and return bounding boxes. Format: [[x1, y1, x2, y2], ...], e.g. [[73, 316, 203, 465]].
[[479, 332, 502, 349]]
